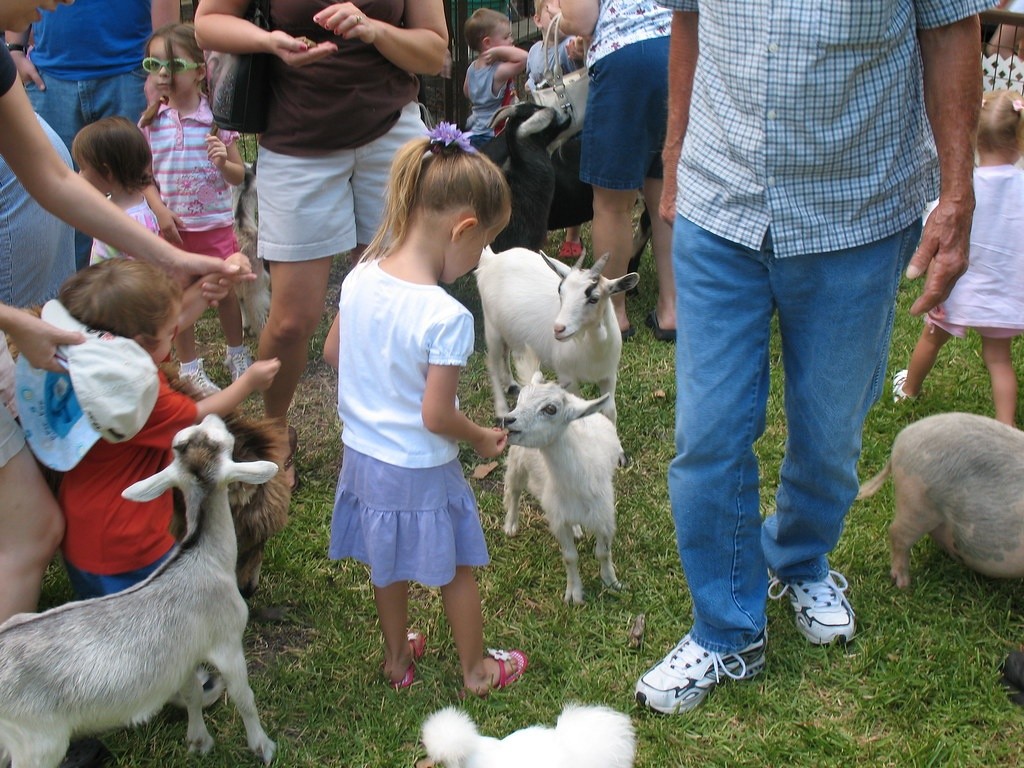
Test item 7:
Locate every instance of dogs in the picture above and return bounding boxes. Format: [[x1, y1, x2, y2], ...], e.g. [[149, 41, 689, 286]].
[[148, 368, 293, 601], [413, 701, 638, 768]]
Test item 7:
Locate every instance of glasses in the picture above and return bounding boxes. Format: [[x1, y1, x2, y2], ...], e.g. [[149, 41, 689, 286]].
[[142, 58, 201, 73]]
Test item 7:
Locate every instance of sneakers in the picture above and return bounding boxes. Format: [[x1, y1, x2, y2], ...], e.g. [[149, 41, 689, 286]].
[[767, 564, 856, 645], [223, 346, 253, 384], [178, 358, 222, 399], [893, 369, 921, 404], [635, 631, 767, 718]]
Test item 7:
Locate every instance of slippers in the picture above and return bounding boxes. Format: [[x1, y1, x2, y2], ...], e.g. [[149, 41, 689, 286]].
[[621, 326, 635, 340], [389, 629, 425, 688], [647, 307, 676, 340], [460, 648, 527, 701]]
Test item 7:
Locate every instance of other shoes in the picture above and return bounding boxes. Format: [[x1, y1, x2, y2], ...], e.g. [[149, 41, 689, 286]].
[[559, 235, 583, 257]]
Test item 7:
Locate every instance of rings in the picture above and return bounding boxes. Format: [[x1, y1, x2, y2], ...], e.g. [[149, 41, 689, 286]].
[[355, 14, 362, 24]]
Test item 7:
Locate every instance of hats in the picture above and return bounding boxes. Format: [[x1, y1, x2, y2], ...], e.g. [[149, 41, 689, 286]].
[[15, 297, 160, 472]]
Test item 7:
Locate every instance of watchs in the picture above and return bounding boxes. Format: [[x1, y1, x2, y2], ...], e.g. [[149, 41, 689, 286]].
[[6, 41, 29, 54]]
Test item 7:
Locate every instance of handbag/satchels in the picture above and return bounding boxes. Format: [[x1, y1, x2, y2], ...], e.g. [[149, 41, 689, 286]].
[[202, 3, 273, 133], [530, 15, 590, 143]]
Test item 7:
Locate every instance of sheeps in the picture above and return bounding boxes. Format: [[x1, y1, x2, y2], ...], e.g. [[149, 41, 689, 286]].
[[0, 413, 279, 768], [850, 412, 1024, 592], [231, 158, 272, 336], [470, 243, 641, 606], [488, 103, 652, 299]]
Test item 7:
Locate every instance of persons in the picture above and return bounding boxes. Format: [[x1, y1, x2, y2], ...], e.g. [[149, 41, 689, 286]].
[[139, 22, 254, 402], [195, 0, 450, 491], [891, 90, 1024, 429], [0, 0, 239, 626], [636, 0, 1001, 716], [4, 0, 182, 272], [69, 118, 159, 268], [547, 0, 680, 343], [323, 125, 525, 697], [57, 256, 281, 714], [985, 0, 1024, 80], [0, 112, 78, 312], [463, 0, 590, 256]]
